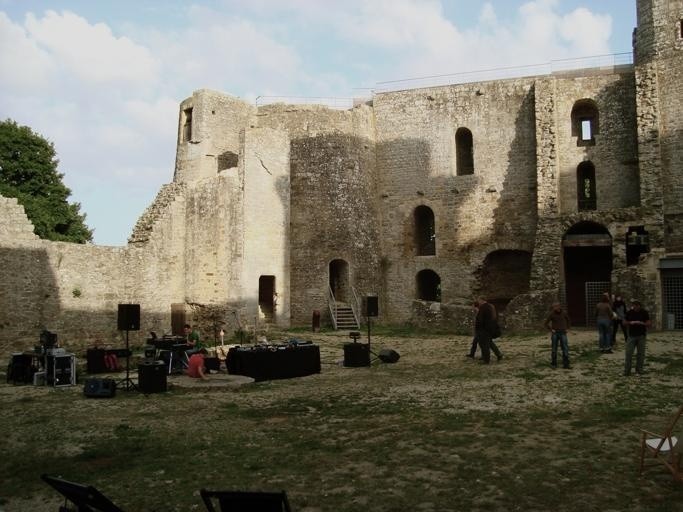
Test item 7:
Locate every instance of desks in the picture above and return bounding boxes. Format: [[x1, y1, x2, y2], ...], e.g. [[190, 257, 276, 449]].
[[229, 343, 321, 382]]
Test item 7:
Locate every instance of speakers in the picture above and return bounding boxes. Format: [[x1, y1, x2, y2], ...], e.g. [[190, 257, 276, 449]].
[[118, 304, 140, 331], [139, 360, 167, 394], [361, 296, 378, 316], [84, 378, 116, 398], [344, 344, 369, 367], [379, 349, 400, 363]]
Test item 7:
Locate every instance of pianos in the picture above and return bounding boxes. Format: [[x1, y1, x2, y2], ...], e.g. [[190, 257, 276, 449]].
[[146, 335, 193, 351]]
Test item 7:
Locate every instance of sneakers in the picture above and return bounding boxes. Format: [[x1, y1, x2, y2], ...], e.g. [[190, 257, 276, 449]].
[[605, 350, 612, 353], [563, 363, 573, 369]]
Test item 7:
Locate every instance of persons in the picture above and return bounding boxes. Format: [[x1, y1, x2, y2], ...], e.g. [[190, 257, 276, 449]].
[[593, 292, 613, 354], [609, 293, 629, 344], [463, 301, 484, 357], [186, 349, 210, 381], [472, 294, 504, 364], [622, 300, 652, 376], [543, 301, 573, 368], [176, 324, 200, 367]]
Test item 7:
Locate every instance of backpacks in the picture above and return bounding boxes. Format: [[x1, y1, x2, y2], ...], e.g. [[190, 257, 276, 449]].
[[103, 352, 121, 373]]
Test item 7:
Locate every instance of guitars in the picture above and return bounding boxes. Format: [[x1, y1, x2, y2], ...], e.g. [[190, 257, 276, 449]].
[[217, 328, 228, 362]]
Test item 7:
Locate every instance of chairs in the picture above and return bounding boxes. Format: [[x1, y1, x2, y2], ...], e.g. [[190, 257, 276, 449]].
[[640, 406, 683, 485]]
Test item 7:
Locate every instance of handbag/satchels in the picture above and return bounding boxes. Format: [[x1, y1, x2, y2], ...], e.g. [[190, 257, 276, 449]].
[[490, 323, 501, 339]]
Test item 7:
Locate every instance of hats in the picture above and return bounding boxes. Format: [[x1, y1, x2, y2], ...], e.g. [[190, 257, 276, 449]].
[[630, 298, 641, 307]]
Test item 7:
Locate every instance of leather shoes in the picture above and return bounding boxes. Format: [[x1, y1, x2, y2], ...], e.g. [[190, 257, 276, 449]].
[[465, 353, 503, 365], [636, 369, 644, 374]]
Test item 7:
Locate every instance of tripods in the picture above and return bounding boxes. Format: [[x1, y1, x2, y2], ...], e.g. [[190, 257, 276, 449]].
[[116, 330, 140, 392], [368, 316, 379, 364]]
